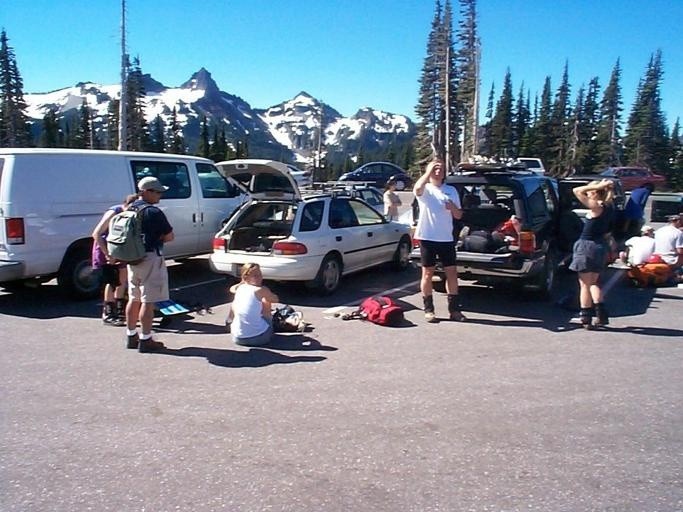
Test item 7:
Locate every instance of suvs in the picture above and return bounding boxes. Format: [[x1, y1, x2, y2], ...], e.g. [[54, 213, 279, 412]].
[[408, 162, 615, 297]]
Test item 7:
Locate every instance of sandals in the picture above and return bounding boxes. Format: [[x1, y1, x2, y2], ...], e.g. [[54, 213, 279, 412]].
[[342, 309, 367, 319]]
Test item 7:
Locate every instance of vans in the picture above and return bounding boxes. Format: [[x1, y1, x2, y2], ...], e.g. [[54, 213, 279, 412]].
[[0, 147, 254, 302]]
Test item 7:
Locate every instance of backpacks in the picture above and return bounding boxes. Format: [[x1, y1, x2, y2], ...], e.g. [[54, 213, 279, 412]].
[[105, 205, 151, 261]]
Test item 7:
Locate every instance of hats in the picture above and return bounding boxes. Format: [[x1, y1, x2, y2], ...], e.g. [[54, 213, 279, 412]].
[[138, 177, 169, 192]]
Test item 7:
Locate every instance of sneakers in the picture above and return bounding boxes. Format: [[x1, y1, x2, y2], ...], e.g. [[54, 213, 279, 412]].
[[127, 332, 163, 352]]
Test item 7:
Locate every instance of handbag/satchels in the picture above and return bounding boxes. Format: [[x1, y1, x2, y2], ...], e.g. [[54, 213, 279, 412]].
[[272, 304, 307, 332], [627, 263, 675, 287], [91, 237, 108, 271], [363, 295, 404, 326], [464, 230, 490, 253]]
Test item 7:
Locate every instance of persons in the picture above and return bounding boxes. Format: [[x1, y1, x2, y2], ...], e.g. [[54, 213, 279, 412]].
[[381, 176, 402, 222], [413, 157, 470, 322], [655, 214, 682, 283], [570, 177, 618, 329], [621, 183, 654, 232], [223, 263, 279, 347], [624, 223, 654, 267], [90, 193, 137, 325], [123, 175, 174, 352]]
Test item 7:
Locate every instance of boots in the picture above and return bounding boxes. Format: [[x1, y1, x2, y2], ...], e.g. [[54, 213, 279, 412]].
[[422, 296, 436, 321], [102, 298, 126, 325], [448, 295, 466, 321], [586, 303, 609, 329], [568, 308, 592, 325]]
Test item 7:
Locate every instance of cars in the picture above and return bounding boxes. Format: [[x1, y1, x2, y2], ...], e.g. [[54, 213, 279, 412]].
[[514, 158, 546, 175], [561, 174, 626, 209], [208, 158, 413, 296], [286, 163, 313, 188], [338, 162, 412, 191], [599, 166, 667, 194]]
